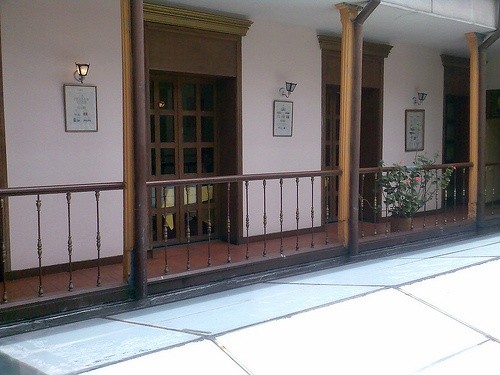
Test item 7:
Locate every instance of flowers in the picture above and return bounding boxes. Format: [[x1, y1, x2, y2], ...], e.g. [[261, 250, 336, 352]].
[[370, 154, 457, 216]]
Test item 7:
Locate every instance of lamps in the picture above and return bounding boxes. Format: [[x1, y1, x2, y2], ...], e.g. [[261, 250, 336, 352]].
[[411, 92, 428, 104], [279, 82, 298, 98], [73, 62, 91, 83]]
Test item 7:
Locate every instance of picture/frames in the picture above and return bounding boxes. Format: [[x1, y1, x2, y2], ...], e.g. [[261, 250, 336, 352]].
[[405, 109, 425, 151], [63, 84, 99, 133], [272, 100, 294, 137]]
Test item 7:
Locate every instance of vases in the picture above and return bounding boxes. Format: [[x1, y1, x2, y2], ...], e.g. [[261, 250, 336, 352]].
[[389, 214, 412, 234]]
